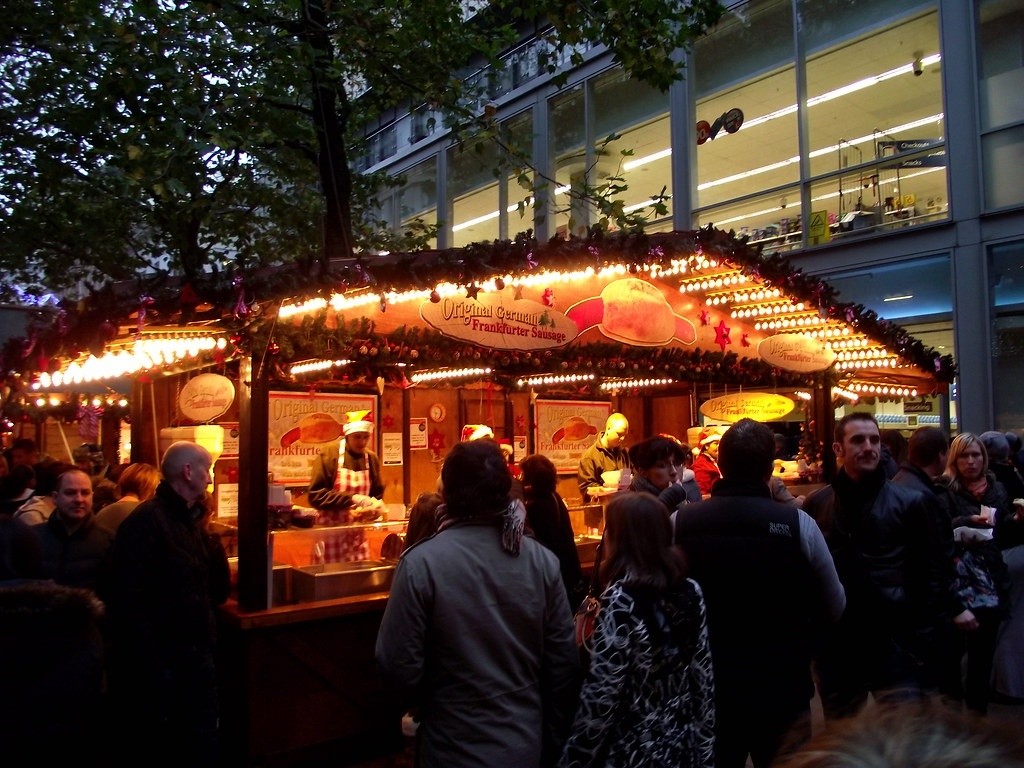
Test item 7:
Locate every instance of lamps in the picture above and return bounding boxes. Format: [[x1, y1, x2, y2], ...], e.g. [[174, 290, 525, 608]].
[[623, 110, 944, 214], [701, 166, 946, 229], [450, 53, 942, 232]]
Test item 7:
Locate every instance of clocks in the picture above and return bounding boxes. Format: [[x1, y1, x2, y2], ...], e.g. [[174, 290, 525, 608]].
[[429, 403, 446, 423]]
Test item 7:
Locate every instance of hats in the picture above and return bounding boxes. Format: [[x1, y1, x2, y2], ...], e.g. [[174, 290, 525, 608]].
[[498, 436, 514, 456], [693, 427, 721, 455], [980, 430, 1008, 463], [343, 410, 374, 435], [460, 423, 495, 443]]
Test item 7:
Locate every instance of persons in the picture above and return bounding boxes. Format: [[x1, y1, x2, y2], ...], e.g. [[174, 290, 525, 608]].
[[977, 429, 1024, 524], [374, 438, 580, 768], [692, 427, 726, 499], [556, 492, 716, 768], [405, 493, 449, 550], [0, 431, 161, 555], [28, 469, 116, 606], [882, 197, 895, 211], [308, 407, 385, 563], [680, 444, 702, 504], [890, 426, 979, 713], [935, 432, 1014, 709], [579, 412, 634, 536], [626, 435, 686, 541], [515, 454, 581, 612], [801, 411, 929, 726], [769, 469, 804, 511], [0, 584, 109, 768], [436, 424, 493, 492], [879, 429, 910, 469], [772, 433, 789, 460], [772, 698, 1023, 768], [670, 418, 849, 767], [109, 441, 229, 767]]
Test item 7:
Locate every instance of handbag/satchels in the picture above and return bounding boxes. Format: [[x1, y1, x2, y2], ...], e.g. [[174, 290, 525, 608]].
[[571, 594, 603, 667], [948, 547, 997, 614]]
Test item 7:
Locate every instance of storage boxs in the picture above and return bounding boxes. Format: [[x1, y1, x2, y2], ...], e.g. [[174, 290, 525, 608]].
[[227, 555, 294, 607], [575, 536, 602, 564], [289, 559, 397, 604]]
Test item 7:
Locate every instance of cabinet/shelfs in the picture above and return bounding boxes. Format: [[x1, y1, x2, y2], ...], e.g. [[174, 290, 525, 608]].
[[746, 205, 915, 256]]
